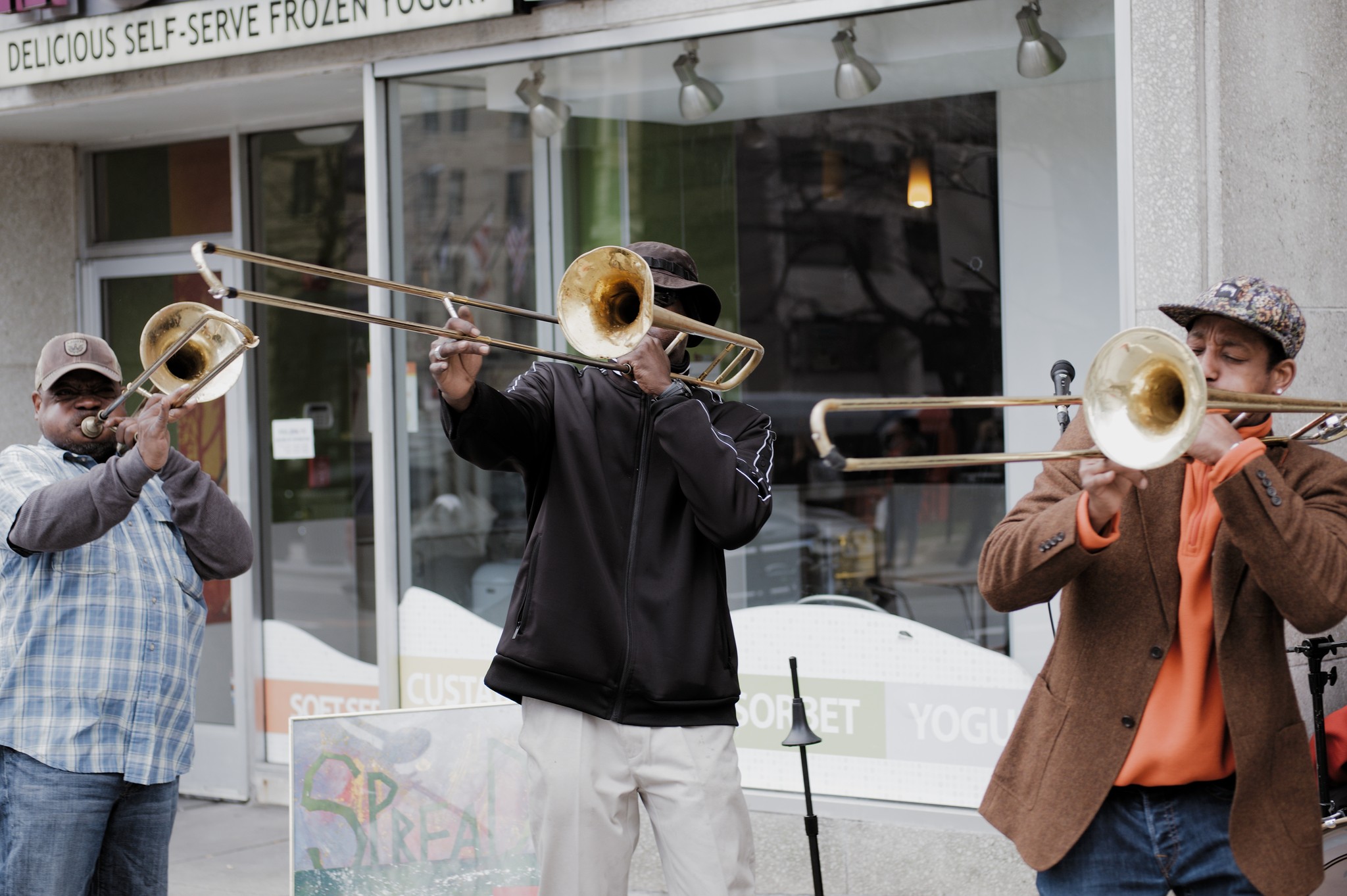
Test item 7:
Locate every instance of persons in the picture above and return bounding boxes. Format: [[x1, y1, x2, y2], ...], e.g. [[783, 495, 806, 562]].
[[0, 333, 255, 896], [977, 278, 1347, 896], [429, 241, 776, 896]]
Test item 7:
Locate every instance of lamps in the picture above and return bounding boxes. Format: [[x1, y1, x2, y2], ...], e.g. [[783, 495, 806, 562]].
[[821, 145, 846, 200], [744, 118, 769, 148], [1010, 0, 1068, 81], [828, 18, 878, 102], [517, 62, 571, 140], [907, 154, 932, 209], [670, 41, 723, 121]]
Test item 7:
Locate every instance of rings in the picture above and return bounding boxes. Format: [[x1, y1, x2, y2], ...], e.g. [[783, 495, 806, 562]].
[[434, 345, 448, 361]]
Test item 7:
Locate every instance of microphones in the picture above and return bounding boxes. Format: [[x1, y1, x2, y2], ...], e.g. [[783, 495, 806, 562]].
[[1050, 359, 1075, 425]]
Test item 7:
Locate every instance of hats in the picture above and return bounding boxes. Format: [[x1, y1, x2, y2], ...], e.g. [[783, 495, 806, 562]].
[[35, 332, 124, 393], [1156, 275, 1305, 357], [622, 240, 721, 347]]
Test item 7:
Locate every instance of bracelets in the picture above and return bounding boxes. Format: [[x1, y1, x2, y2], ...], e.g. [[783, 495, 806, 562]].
[[1229, 441, 1243, 450]]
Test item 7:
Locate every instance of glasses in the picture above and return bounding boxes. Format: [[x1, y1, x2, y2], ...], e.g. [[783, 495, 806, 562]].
[[654, 289, 679, 308]]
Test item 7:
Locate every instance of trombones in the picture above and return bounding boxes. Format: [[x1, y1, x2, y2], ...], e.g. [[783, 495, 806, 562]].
[[807, 327, 1347, 475], [80, 300, 260, 458], [188, 238, 765, 393]]
